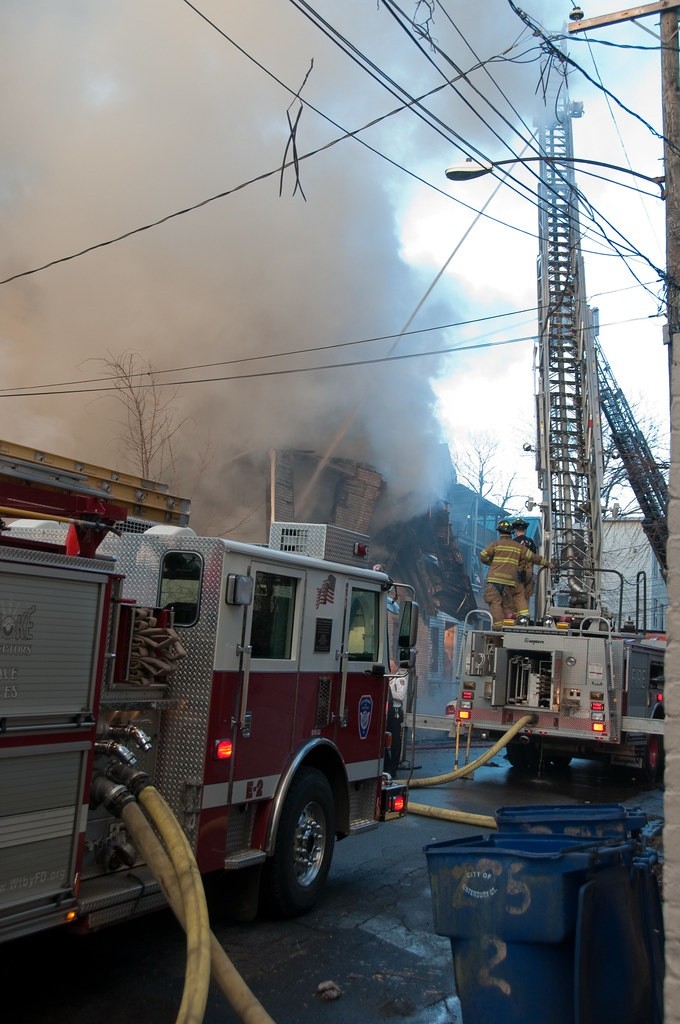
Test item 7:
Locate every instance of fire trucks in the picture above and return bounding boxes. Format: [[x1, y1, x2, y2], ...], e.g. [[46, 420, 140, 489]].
[[452, 7, 665, 794], [1, 440, 420, 948]]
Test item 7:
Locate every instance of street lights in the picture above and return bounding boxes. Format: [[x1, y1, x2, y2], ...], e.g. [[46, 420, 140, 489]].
[[443, 155, 679, 1024]]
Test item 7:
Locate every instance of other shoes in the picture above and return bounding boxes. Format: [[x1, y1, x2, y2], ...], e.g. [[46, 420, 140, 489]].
[[386, 771, 397, 777]]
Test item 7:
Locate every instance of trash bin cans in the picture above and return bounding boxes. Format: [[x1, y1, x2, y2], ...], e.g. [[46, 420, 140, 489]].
[[421, 802, 668, 1024]]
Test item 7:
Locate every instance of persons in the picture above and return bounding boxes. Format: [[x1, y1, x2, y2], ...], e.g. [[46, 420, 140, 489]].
[[389, 658, 409, 768], [479, 519, 553, 622]]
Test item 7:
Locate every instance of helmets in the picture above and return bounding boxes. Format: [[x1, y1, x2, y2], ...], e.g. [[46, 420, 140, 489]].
[[495, 520, 512, 533], [512, 519, 529, 529]]
